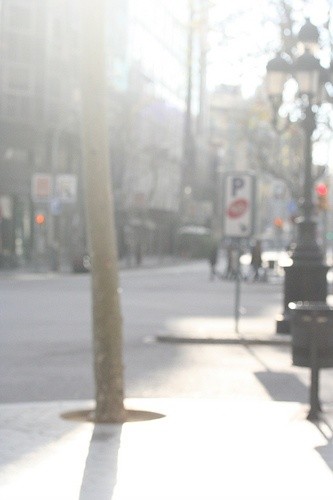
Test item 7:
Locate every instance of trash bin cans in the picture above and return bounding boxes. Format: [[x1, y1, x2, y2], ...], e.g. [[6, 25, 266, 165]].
[[269, 260, 275, 268]]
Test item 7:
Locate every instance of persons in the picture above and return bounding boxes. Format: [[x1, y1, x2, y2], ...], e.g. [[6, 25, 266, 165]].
[[204, 233, 267, 282]]
